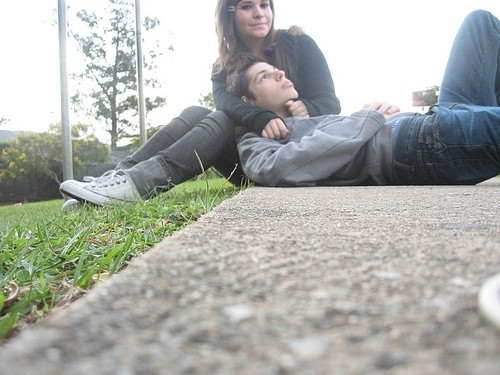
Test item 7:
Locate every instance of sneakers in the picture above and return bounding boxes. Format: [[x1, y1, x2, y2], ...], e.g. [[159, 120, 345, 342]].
[[60, 170, 146, 208], [60, 198, 82, 213]]
